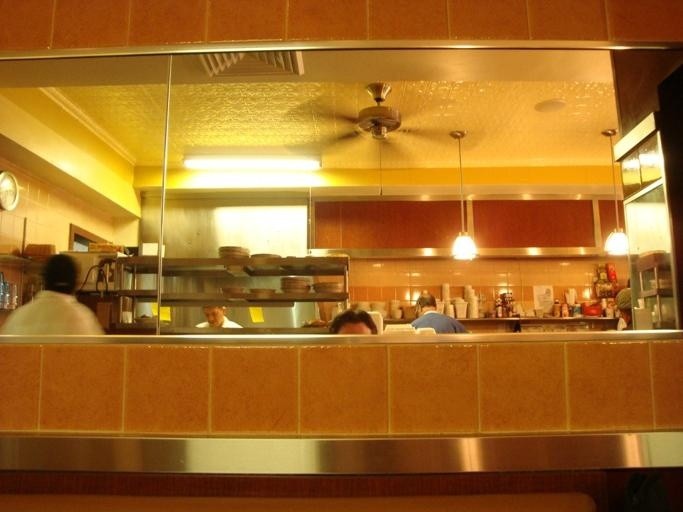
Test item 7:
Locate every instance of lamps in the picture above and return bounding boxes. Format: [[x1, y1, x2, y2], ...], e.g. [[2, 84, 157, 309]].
[[602, 129, 628, 256], [449, 129, 478, 261]]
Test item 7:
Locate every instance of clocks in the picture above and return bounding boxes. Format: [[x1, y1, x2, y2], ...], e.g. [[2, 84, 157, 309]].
[[0, 171, 19, 210]]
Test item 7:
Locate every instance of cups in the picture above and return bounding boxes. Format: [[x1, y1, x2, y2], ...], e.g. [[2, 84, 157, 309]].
[[348, 282, 480, 320]]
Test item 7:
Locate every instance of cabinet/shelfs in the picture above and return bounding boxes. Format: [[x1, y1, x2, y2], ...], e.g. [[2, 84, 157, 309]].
[[636, 253, 673, 329], [114, 256, 350, 334], [1, 254, 43, 311]]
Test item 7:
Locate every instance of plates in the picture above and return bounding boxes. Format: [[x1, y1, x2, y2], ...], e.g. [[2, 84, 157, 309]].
[[214, 244, 346, 299]]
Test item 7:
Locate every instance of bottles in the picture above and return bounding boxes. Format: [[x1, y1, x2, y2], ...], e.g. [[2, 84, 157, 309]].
[[552, 300, 582, 318]]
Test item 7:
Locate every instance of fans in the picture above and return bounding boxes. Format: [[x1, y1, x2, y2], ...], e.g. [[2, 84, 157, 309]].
[[282, 84, 478, 155]]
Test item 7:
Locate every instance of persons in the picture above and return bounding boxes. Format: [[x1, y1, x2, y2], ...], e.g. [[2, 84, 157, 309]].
[[616, 288, 634, 331], [407, 290, 467, 335], [326, 306, 376, 338], [0, 250, 109, 335], [191, 303, 246, 329]]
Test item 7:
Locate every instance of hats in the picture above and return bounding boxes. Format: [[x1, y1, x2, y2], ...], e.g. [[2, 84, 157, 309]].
[[42, 254, 77, 287]]
